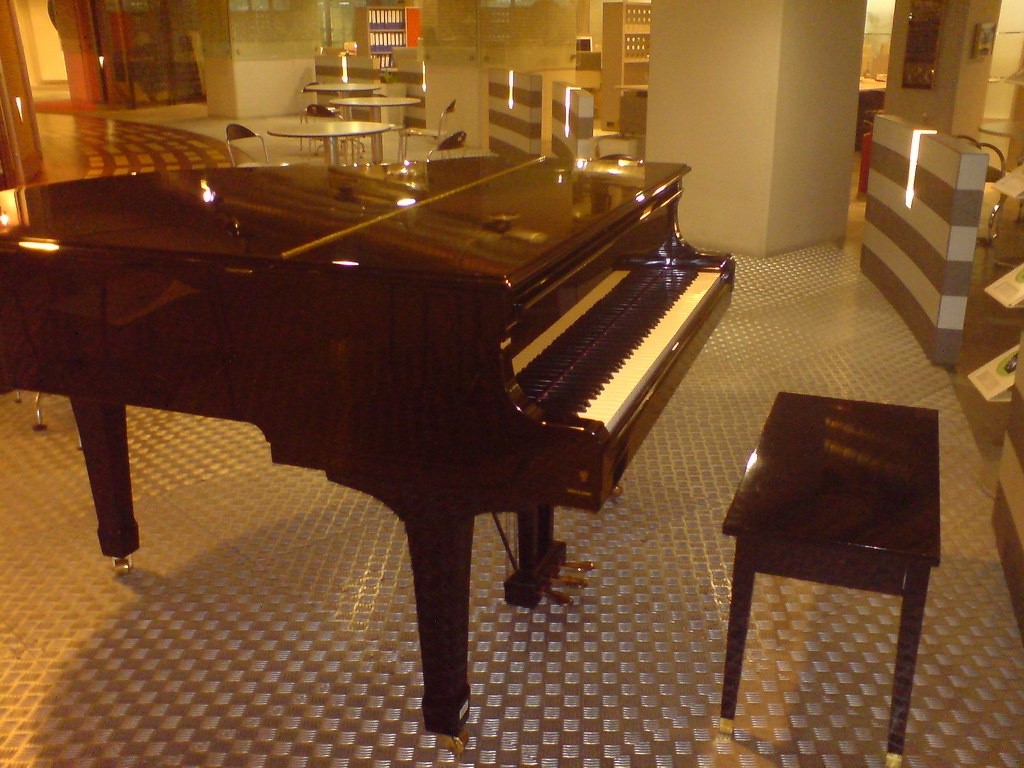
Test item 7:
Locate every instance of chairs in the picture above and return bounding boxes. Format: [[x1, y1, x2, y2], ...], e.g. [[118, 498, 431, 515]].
[[224, 82, 466, 162], [953, 135, 1009, 249]]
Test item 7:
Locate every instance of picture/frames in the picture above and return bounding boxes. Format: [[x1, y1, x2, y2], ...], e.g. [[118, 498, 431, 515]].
[[901, 0, 947, 90]]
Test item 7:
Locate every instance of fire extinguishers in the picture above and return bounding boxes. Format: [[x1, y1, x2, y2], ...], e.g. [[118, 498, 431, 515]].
[[858, 119, 873, 192]]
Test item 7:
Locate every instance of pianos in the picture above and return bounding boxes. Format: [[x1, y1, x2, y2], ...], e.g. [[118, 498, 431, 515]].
[[0, 152, 738, 739]]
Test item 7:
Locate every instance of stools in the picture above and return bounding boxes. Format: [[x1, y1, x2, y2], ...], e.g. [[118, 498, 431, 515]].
[[718, 392, 943, 767]]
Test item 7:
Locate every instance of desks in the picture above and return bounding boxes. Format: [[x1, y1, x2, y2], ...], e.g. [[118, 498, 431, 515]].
[[332, 96, 421, 162], [977, 119, 1024, 172], [270, 122, 390, 169], [307, 82, 381, 119]]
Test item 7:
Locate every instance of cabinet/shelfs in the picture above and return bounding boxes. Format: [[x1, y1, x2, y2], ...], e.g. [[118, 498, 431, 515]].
[[354, 4, 407, 74]]
